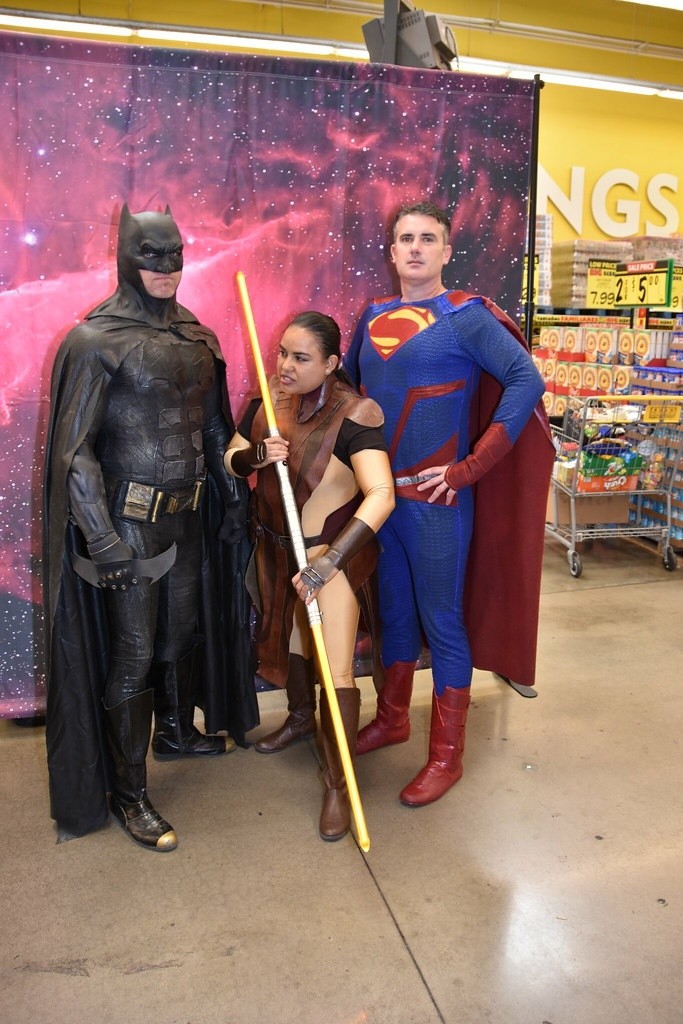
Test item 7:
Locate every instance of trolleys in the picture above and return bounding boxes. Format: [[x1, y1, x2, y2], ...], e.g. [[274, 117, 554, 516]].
[[547, 394, 683, 579]]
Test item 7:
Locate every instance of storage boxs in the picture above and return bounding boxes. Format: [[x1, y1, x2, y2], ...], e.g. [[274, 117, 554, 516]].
[[532, 324, 683, 550]]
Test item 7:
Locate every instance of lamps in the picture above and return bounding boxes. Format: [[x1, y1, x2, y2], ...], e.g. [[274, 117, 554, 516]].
[[361, 0, 457, 72]]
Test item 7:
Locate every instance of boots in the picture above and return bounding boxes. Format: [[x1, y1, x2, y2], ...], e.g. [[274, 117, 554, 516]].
[[101, 688, 178, 852], [319, 687, 362, 843], [398, 681, 472, 808], [254, 652, 318, 754], [151, 643, 238, 763], [355, 653, 419, 757]]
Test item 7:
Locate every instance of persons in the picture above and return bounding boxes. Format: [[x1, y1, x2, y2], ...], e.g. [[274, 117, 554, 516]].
[[225, 308, 398, 843], [60, 200, 246, 857], [337, 202, 547, 806]]
